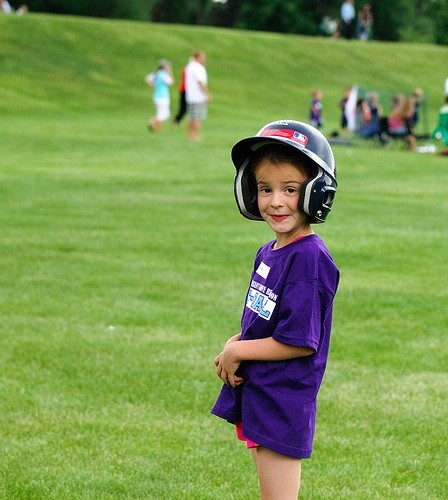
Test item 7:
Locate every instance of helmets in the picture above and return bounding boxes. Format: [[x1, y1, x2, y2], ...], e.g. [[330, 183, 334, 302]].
[[231, 120, 338, 224]]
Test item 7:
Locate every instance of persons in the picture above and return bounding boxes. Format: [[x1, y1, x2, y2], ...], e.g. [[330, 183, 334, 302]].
[[144, 49, 210, 141], [211, 120, 341, 500], [311, 89, 322, 129], [331, 0, 373, 42], [339, 79, 448, 155]]
